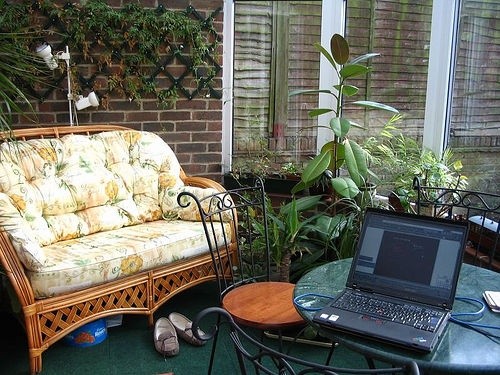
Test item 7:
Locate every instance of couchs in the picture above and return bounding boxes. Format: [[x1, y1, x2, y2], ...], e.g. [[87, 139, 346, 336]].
[[0, 123, 242, 374]]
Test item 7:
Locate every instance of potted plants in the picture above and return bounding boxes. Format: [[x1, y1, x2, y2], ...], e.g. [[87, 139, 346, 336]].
[[380, 133, 469, 219], [281, 29, 400, 263], [240, 188, 321, 282], [351, 114, 402, 207]]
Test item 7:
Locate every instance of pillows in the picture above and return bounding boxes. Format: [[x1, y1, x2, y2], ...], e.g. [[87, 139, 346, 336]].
[[161, 187, 232, 223], [0, 193, 47, 274]]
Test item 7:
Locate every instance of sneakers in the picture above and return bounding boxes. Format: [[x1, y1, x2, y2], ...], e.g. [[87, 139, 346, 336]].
[[169, 311, 207, 346], [154, 316, 180, 362]]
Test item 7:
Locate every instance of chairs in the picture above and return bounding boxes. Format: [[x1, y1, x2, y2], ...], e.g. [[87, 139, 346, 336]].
[[413, 175, 500, 272], [177, 172, 305, 375], [192, 307, 419, 375]]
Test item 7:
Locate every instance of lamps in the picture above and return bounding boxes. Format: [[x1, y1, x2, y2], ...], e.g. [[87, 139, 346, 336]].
[[35, 43, 59, 72], [75, 91, 101, 111]]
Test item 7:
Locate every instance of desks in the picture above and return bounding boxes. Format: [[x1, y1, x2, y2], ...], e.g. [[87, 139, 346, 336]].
[[293, 256, 499, 374]]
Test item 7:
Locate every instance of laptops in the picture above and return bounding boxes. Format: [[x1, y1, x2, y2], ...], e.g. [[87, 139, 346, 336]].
[[310, 205, 471, 355]]
